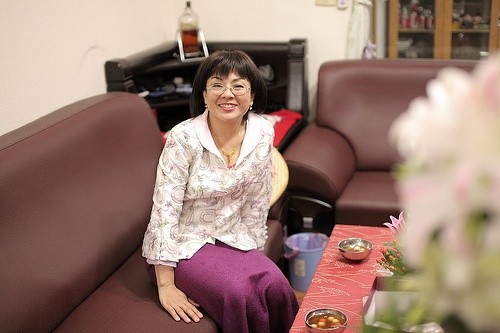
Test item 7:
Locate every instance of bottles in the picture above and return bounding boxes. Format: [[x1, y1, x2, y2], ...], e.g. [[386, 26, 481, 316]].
[[179, 0, 200, 56]]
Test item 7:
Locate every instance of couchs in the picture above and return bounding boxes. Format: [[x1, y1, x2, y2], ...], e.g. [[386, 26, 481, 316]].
[[281, 59, 485, 237], [0, 92, 285, 333]]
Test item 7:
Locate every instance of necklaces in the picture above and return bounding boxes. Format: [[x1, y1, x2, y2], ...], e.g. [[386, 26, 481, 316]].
[[211, 126, 241, 162]]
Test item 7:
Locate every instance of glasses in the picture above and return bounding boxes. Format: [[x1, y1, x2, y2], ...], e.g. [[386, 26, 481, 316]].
[[206, 82, 252, 95]]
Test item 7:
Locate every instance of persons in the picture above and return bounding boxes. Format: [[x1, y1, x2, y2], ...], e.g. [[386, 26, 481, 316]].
[[141, 49, 299, 332]]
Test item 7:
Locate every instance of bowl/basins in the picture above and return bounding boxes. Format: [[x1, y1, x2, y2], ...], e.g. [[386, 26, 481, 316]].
[[338, 238, 373, 261], [304, 308, 348, 333]]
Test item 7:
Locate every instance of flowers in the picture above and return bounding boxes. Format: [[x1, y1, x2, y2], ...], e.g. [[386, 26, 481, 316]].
[[375, 211, 408, 283]]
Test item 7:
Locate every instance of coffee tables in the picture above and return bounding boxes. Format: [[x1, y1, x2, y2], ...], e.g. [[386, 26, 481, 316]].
[[291, 224, 397, 333]]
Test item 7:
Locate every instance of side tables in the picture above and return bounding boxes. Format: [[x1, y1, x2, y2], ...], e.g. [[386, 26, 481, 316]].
[[104, 38, 308, 154]]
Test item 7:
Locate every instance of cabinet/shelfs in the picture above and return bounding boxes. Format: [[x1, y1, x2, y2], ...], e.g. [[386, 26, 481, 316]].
[[386, 1, 500, 60]]
